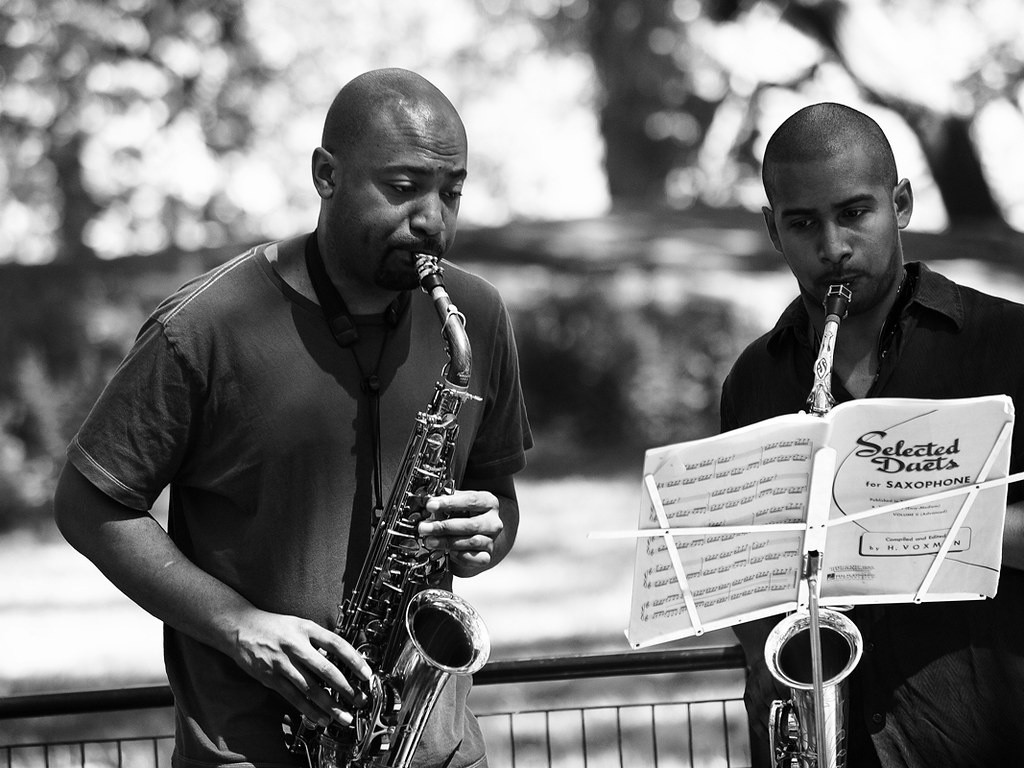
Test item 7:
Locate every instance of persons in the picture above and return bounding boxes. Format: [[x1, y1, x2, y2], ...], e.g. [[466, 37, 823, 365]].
[[55, 66, 536, 768], [720, 103, 1024, 768]]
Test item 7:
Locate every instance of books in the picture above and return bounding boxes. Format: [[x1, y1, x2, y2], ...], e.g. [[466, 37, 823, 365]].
[[628, 394, 1015, 647]]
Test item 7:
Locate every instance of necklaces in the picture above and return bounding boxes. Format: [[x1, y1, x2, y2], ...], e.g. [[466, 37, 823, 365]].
[[813, 268, 915, 398]]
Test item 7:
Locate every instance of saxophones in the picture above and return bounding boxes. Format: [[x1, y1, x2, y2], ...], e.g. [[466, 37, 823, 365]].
[[282, 252, 493, 768], [762, 279, 866, 768]]
